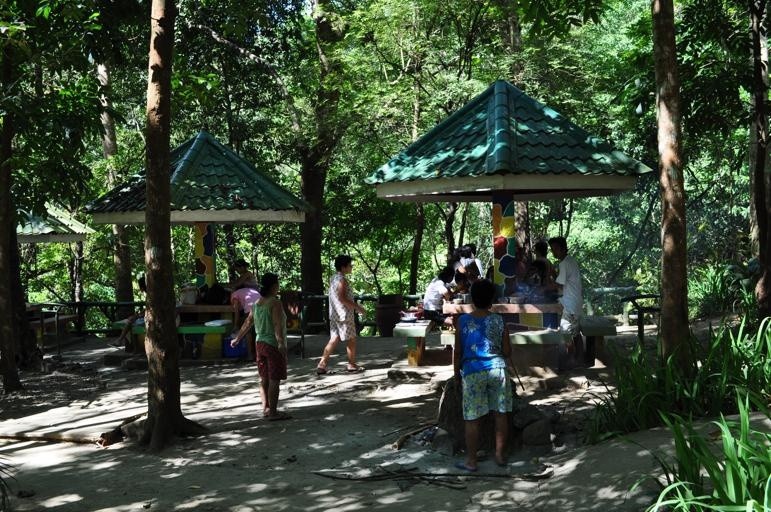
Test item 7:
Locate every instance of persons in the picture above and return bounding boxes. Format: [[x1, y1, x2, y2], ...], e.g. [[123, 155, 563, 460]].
[[446, 244, 481, 279], [549, 238, 582, 329], [230, 274, 294, 420], [231, 284, 262, 364], [533, 242, 548, 261], [107, 276, 144, 351], [454, 280, 512, 472], [423, 267, 454, 324], [231, 259, 261, 288], [315, 256, 366, 374]]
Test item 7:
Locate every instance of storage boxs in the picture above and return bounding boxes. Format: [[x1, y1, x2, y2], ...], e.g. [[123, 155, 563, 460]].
[[224, 335, 248, 357]]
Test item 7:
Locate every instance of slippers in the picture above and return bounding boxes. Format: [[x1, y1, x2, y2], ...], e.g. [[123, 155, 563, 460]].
[[455, 461, 477, 472], [318, 368, 335, 374], [348, 366, 365, 371], [263, 411, 277, 416], [495, 454, 505, 466], [269, 414, 291, 420]]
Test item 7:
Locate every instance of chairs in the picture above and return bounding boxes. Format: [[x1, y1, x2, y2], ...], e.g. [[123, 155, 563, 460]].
[[280, 290, 304, 360]]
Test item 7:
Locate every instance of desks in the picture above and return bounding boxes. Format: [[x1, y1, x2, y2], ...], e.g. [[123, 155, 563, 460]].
[[442, 302, 564, 330], [176, 304, 236, 321], [26, 302, 44, 320]]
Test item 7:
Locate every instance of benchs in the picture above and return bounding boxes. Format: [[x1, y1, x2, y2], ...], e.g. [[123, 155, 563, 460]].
[[132, 325, 232, 359], [579, 316, 617, 359], [440, 330, 562, 375], [41, 310, 65, 317], [32, 314, 77, 343], [112, 319, 145, 352], [393, 320, 434, 367]]
